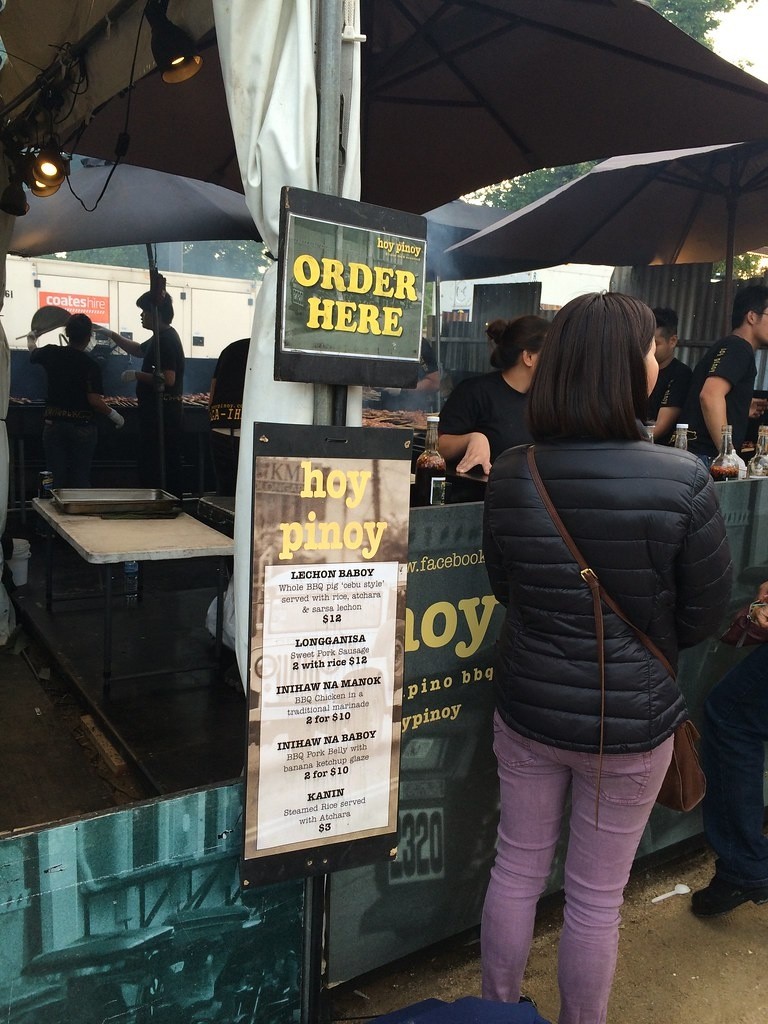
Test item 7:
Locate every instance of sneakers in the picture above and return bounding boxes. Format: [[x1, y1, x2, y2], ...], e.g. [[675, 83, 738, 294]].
[[692, 876, 768, 915]]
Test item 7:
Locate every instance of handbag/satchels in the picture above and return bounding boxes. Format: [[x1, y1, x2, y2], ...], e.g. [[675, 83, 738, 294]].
[[721, 581, 768, 649], [657, 720, 707, 813]]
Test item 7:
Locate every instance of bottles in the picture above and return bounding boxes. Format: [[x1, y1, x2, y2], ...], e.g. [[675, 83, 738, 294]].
[[746, 425, 768, 480], [710, 424, 745, 481], [413, 417, 446, 507], [124, 560, 139, 599], [644, 421, 655, 444], [675, 423, 689, 452]]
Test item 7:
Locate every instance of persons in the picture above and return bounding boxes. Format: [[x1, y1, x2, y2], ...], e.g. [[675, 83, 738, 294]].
[[90, 288, 186, 488], [437, 314, 553, 476], [644, 305, 690, 444], [692, 559, 768, 917], [29, 314, 125, 489], [685, 285, 768, 456], [480, 291, 737, 1024], [402, 335, 442, 395]]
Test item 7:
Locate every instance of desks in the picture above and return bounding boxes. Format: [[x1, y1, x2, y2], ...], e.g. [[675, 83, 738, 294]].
[[32, 497, 235, 693]]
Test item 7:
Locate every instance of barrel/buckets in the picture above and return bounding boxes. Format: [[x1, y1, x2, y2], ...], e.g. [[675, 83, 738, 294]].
[[4, 538, 31, 586]]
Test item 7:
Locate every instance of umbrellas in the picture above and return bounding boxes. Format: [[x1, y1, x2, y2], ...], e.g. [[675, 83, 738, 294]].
[[0, 150, 264, 485], [421, 198, 566, 283], [0, 0, 768, 426], [445, 141, 768, 338]]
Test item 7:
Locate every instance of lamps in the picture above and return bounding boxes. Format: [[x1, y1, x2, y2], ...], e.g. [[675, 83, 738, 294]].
[[145, 3, 203, 83], [0, 176, 30, 216], [5, 133, 66, 197]]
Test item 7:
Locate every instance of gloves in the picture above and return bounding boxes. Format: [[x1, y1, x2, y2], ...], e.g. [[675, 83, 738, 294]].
[[27, 329, 38, 341], [121, 370, 136, 383], [107, 408, 124, 429]]
